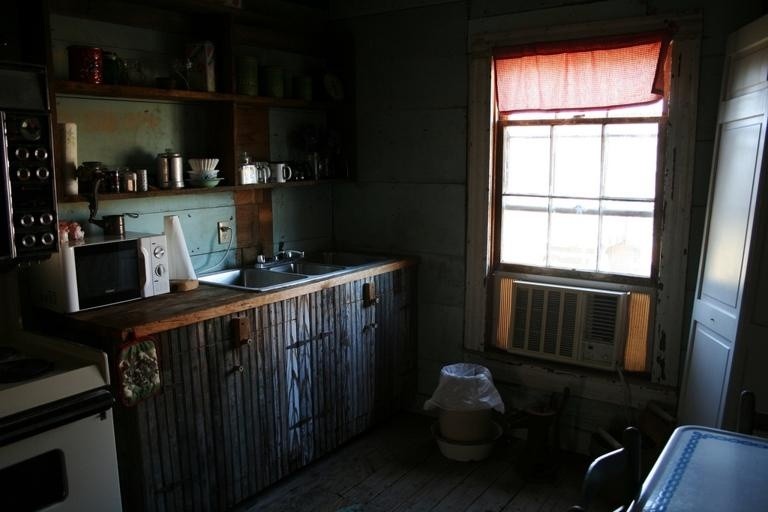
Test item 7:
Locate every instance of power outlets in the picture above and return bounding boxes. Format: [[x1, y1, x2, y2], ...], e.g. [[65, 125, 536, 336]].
[[218, 221, 233, 243]]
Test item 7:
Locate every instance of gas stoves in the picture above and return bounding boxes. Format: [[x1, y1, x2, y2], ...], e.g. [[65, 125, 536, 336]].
[[0, 335, 107, 421]]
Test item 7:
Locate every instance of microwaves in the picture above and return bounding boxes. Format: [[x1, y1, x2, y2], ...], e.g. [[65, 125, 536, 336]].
[[18, 229, 172, 317]]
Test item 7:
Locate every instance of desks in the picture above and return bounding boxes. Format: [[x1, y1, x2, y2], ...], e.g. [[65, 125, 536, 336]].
[[625, 425, 768, 512]]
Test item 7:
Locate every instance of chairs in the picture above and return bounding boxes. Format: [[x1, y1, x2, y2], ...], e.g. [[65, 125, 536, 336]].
[[738, 390, 768, 436], [569, 427, 641, 512]]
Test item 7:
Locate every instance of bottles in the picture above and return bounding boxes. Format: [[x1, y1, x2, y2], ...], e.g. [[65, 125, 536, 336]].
[[106, 168, 148, 193]]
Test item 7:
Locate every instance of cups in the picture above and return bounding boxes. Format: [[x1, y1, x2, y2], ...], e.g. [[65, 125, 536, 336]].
[[252, 160, 293, 184]]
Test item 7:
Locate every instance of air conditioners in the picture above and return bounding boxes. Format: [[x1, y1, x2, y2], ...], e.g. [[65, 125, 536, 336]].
[[489, 272, 658, 375]]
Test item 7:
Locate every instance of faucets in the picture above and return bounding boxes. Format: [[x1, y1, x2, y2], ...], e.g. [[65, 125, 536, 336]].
[[271, 249, 302, 262]]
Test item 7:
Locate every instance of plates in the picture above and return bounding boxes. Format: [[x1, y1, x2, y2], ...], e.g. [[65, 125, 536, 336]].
[[185, 177, 225, 188]]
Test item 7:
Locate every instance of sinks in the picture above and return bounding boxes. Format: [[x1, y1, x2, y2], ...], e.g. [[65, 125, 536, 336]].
[[265, 259, 345, 280], [195, 266, 309, 292]]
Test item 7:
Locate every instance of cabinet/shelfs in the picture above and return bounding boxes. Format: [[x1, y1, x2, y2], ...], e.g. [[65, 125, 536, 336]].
[[678, 13, 768, 431], [49, 81, 351, 204], [127, 262, 419, 512]]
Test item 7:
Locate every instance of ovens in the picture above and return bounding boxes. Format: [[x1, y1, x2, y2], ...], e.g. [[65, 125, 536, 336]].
[[0, 385, 124, 512]]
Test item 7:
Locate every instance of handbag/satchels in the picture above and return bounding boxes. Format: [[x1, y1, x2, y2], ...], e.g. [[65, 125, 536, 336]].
[[100, 51, 195, 88]]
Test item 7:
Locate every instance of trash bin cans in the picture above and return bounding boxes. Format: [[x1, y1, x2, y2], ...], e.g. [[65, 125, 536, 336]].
[[439, 363, 492, 443]]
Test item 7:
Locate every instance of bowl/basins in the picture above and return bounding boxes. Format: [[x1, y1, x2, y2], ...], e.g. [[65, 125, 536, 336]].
[[187, 169, 219, 179]]
